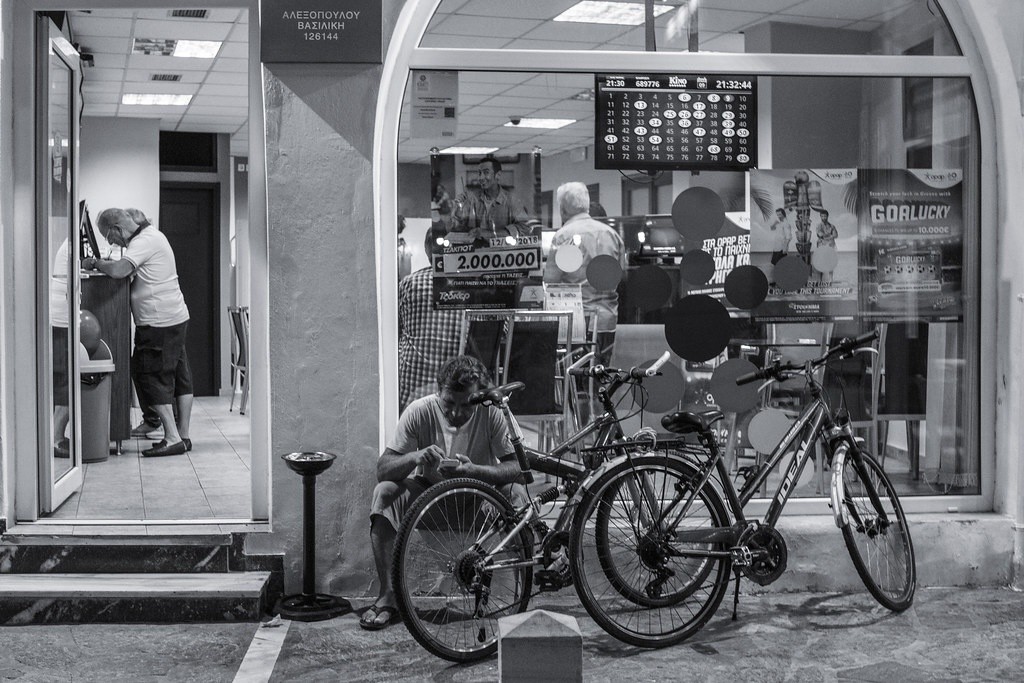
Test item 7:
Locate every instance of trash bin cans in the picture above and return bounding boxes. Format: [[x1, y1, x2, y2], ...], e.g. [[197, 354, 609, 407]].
[[79, 309, 115, 463]]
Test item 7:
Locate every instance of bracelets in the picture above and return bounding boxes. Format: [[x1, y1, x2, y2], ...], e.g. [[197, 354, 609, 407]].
[[494, 232, 497, 238]]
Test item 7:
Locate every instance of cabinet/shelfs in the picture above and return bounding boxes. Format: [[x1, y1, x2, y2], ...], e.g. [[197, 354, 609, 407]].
[[79, 275, 131, 456]]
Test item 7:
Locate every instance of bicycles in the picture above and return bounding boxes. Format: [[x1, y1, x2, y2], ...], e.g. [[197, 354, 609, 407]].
[[387, 348, 726, 667], [559, 328, 919, 649]]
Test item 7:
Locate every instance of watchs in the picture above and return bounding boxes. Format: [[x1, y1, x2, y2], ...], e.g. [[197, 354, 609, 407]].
[[90, 259, 98, 268]]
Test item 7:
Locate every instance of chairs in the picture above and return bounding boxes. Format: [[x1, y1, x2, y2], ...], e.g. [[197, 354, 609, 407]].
[[458, 308, 574, 483], [227, 305, 249, 415], [716, 320, 928, 497]]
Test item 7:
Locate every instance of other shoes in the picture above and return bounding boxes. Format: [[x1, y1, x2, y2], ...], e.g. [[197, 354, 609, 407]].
[[142, 439, 185, 456], [152, 439, 192, 451]]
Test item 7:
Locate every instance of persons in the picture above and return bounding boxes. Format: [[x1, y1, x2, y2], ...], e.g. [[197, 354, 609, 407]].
[[398, 225, 472, 417], [544, 182, 625, 444], [812, 210, 838, 287], [398, 217, 412, 284], [435, 183, 449, 212], [83, 208, 193, 457], [769, 209, 793, 295], [126, 208, 188, 438], [360, 354, 536, 637], [448, 156, 534, 239]]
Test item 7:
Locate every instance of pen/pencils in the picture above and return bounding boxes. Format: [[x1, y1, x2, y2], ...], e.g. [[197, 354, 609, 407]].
[[461, 177, 465, 192], [107, 250, 112, 259]]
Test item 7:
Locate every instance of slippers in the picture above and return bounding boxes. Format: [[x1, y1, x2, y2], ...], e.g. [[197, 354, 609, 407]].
[[358, 605, 399, 629]]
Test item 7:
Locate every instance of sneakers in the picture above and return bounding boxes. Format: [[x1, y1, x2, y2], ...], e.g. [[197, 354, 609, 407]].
[[145, 423, 165, 439], [131, 420, 156, 435]]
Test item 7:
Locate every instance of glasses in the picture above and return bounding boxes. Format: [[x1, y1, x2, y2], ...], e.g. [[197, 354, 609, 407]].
[[104, 224, 111, 242]]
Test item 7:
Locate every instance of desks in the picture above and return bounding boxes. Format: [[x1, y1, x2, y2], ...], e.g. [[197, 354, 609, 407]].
[[724, 338, 885, 498]]
